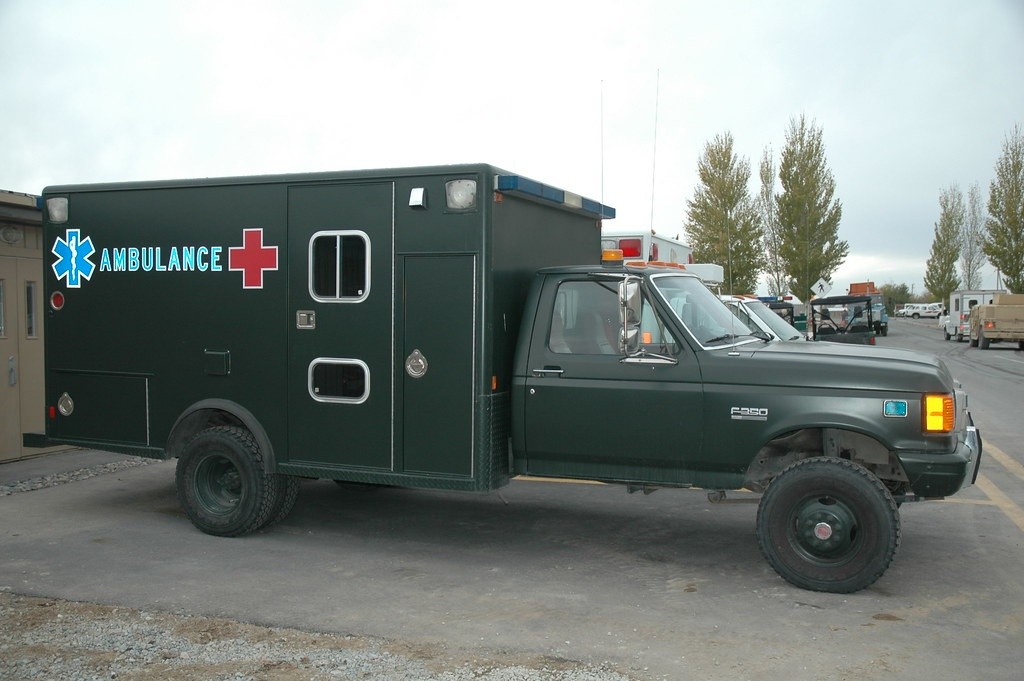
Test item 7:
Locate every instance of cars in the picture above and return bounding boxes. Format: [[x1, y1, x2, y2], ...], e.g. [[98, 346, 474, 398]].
[[898, 302, 947, 319]]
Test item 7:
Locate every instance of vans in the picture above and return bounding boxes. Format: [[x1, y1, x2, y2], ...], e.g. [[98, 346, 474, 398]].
[[718, 295, 808, 340]]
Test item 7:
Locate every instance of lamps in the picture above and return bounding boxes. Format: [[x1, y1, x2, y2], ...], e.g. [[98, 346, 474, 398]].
[[0, 226, 24, 244]]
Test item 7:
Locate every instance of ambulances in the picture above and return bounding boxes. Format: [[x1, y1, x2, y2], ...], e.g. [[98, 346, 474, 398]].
[[37, 157, 985, 593]]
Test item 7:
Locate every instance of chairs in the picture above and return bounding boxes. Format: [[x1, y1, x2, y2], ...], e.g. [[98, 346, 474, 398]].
[[850, 326, 869, 333], [818, 327, 836, 335], [574, 311, 615, 354], [548, 312, 572, 353]]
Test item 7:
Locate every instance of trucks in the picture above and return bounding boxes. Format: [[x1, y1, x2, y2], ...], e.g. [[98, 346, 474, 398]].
[[601, 232, 808, 341], [847, 280, 889, 336], [938, 289, 1024, 350]]
